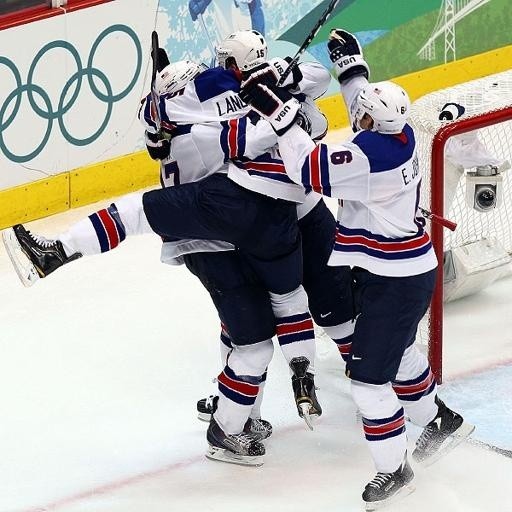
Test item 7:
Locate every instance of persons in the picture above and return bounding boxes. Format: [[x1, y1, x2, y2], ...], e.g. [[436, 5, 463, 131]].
[[1, 27, 359, 462], [238, 27, 466, 507]]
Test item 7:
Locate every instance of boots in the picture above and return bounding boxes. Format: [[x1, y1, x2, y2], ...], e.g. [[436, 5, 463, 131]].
[[243, 417, 272, 441], [206, 396, 266, 457], [360, 450, 415, 502], [289, 356, 322, 417], [413, 394, 463, 464], [195, 394, 219, 414], [12, 223, 83, 278]]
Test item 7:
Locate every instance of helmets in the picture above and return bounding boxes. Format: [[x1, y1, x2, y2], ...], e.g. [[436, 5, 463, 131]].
[[214, 29, 269, 72], [353, 81, 410, 135], [154, 61, 208, 100]]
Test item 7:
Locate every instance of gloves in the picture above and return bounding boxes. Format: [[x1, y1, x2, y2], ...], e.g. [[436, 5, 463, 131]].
[[328, 28, 370, 83], [238, 80, 302, 136], [245, 57, 303, 91], [145, 131, 172, 161]]
